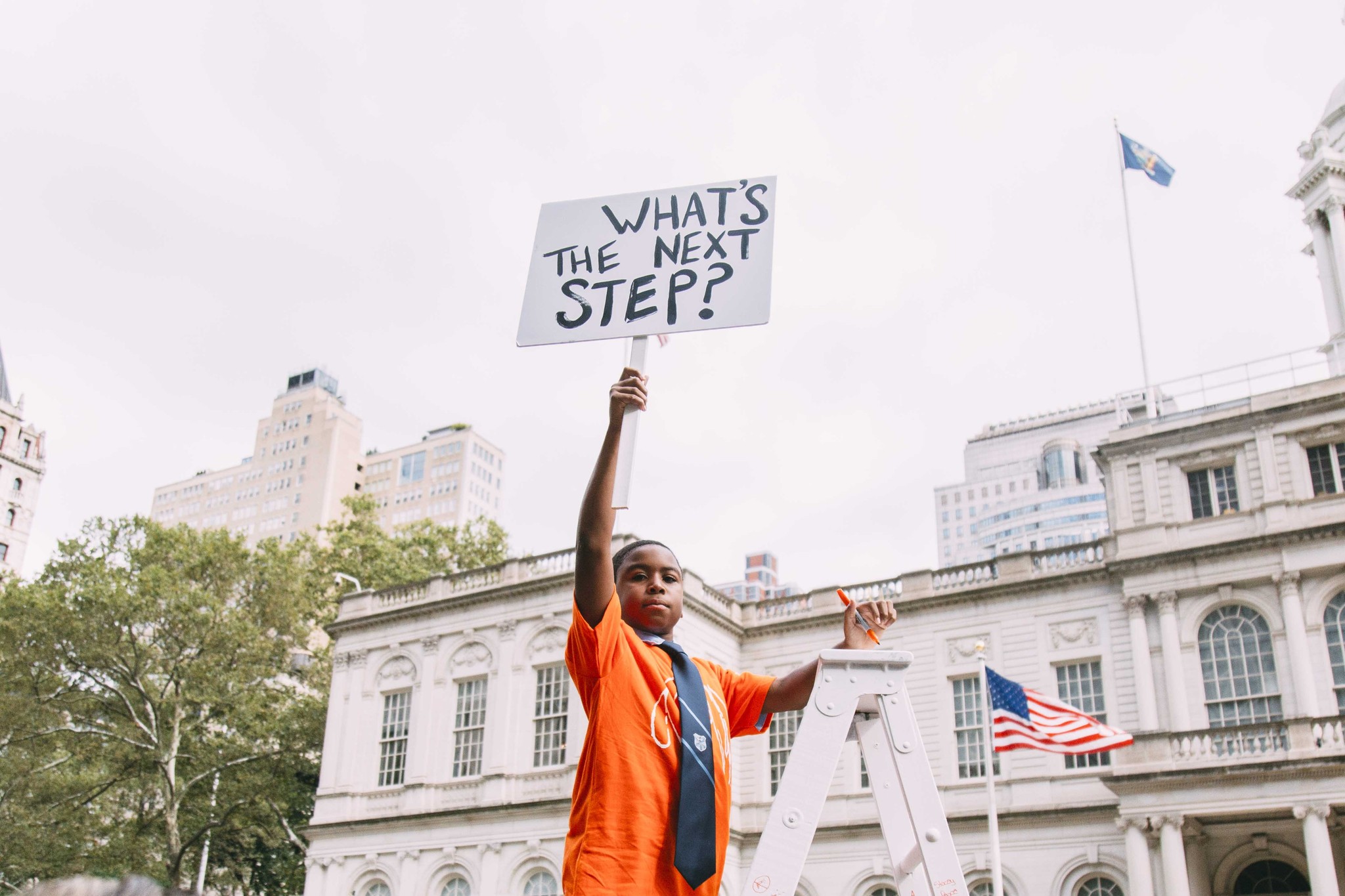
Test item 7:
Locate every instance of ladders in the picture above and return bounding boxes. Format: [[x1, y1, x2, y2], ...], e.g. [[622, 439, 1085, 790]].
[[746, 648, 981, 896]]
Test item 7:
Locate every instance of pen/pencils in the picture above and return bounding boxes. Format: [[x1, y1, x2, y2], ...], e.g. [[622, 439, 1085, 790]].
[[837, 588, 882, 645]]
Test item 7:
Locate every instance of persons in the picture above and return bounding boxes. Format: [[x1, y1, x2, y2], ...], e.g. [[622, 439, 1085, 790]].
[[564, 368, 897, 896]]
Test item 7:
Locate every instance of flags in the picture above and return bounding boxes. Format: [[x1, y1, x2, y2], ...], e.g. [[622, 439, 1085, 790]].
[[1117, 132, 1174, 185], [984, 665, 1134, 754]]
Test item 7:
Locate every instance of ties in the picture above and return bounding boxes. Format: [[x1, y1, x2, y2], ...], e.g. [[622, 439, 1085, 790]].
[[661, 640, 717, 891]]
